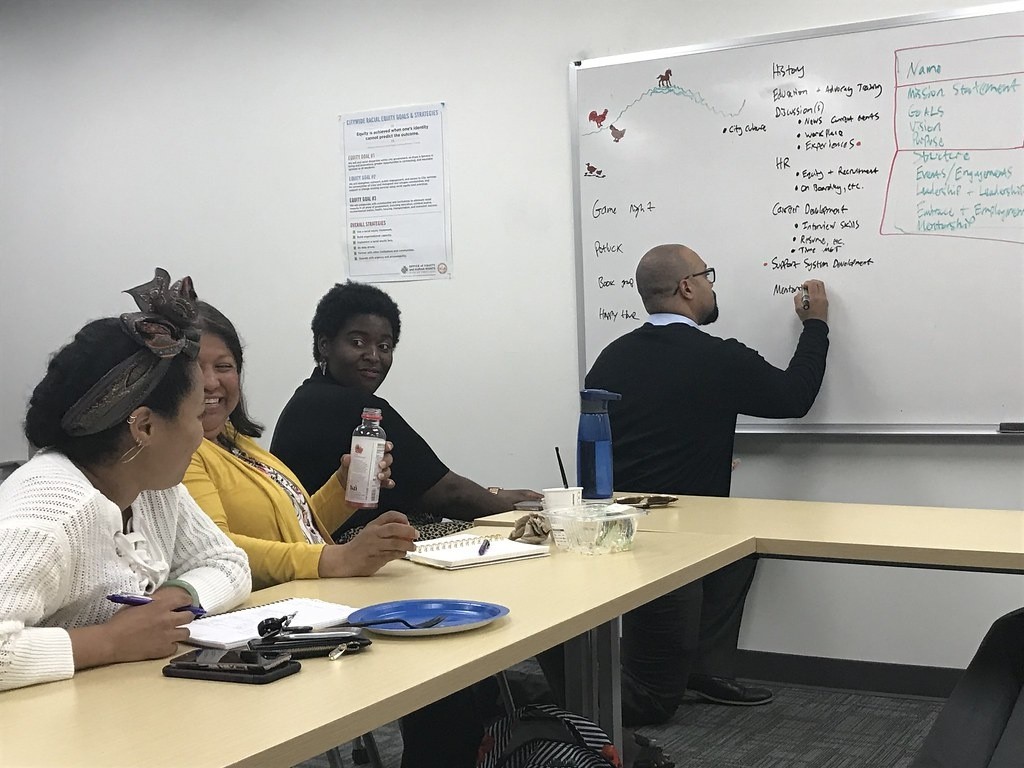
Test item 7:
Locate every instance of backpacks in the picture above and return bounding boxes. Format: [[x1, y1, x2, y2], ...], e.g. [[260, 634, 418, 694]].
[[477, 703, 622, 768]]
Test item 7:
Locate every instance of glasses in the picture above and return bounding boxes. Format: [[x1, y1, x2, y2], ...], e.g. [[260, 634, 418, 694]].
[[675, 267, 717, 297], [614, 495, 677, 507]]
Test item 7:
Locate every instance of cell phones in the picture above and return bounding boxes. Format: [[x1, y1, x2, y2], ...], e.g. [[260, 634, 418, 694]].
[[513, 498, 542, 511], [162, 647, 301, 685], [276, 627, 361, 640]]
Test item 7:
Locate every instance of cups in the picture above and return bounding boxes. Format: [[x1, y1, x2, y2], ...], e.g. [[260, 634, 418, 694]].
[[542, 487, 583, 511]]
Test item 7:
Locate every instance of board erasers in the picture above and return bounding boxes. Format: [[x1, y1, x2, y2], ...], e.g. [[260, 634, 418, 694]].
[[999, 423, 1024, 434]]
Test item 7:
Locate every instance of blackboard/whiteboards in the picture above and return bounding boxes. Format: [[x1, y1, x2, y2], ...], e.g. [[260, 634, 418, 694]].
[[570, 0, 1024, 434]]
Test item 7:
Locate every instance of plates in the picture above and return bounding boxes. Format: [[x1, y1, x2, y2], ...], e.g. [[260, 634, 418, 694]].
[[347, 599, 510, 636], [614, 496, 679, 507]]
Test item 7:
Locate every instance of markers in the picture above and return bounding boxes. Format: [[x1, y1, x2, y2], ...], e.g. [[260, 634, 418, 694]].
[[802, 286, 809, 310]]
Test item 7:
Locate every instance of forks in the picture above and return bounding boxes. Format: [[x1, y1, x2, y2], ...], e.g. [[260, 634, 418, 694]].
[[326, 616, 445, 629]]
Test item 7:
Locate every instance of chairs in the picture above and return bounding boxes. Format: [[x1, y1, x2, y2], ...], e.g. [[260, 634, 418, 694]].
[[911, 608, 1024, 768]]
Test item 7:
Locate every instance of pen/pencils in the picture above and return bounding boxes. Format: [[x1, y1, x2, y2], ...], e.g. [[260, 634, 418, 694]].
[[479, 539, 490, 555], [107, 594, 208, 613]]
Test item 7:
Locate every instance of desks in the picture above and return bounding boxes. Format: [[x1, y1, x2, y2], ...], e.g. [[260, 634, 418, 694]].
[[2, 491, 1024, 768]]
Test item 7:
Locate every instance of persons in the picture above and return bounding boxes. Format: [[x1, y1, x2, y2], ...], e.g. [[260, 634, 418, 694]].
[[580, 245, 830, 726], [181, 301, 420, 592], [0, 267, 253, 690], [268, 281, 545, 543]]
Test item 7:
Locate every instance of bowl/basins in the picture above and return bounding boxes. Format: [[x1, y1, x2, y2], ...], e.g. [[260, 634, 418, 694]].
[[536, 502, 651, 554]]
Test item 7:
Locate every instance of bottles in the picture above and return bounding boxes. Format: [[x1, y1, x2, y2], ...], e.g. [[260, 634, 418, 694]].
[[576, 389, 622, 499], [344, 409, 386, 509]]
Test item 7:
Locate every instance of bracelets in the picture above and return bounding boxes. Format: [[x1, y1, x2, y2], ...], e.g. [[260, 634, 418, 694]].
[[488, 487, 503, 495]]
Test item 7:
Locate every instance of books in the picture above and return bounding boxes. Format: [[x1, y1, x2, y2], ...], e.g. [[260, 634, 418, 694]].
[[405, 533, 551, 571], [177, 597, 360, 650]]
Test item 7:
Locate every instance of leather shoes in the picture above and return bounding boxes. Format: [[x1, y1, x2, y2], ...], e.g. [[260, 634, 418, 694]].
[[681, 673, 774, 704]]
